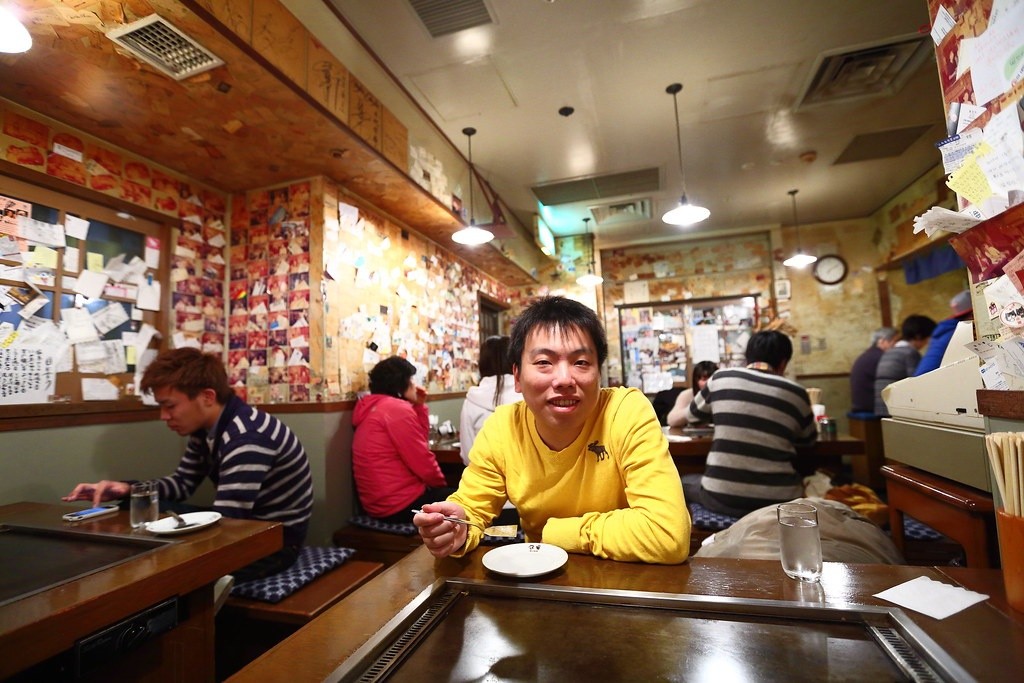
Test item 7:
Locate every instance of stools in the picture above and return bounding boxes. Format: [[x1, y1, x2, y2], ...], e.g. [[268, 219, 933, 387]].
[[688, 503, 960, 561], [334, 509, 425, 569], [212, 549, 382, 629]]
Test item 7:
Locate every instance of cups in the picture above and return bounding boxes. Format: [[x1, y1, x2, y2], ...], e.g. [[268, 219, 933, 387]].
[[778, 503, 822, 584], [429, 424, 437, 448], [820, 418, 836, 435], [811, 405, 825, 433], [130, 481, 159, 528], [997, 506, 1024, 613]]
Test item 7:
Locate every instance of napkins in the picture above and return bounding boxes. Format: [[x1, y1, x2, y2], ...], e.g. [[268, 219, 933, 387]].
[[872, 575, 991, 619]]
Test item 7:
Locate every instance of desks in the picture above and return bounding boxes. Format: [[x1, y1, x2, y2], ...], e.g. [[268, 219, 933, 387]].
[[881, 462, 996, 568], [514, 424, 517, 426], [662, 425, 865, 473], [224, 541, 1024, 683], [429, 430, 464, 465], [0, 501, 284, 683]]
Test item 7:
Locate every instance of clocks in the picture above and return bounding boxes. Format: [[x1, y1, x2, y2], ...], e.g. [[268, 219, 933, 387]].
[[813, 253, 847, 284]]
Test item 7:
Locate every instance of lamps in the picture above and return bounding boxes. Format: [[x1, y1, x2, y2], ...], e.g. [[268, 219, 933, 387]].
[[452, 127, 495, 245], [576, 217, 602, 285], [783, 190, 817, 266], [662, 83, 709, 225]]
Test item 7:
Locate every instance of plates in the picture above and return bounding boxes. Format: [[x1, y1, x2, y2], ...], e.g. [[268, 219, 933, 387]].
[[146, 512, 222, 534], [683, 430, 714, 435], [452, 443, 460, 447], [482, 543, 568, 578]]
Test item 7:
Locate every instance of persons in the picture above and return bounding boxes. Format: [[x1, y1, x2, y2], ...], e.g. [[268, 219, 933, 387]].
[[172, 177, 310, 401], [459, 336, 524, 466], [873, 313, 937, 416], [912, 289, 973, 375], [412, 294, 692, 564], [665, 361, 719, 426], [850, 329, 900, 412], [686, 331, 818, 520], [60, 346, 314, 584], [352, 355, 457, 523]]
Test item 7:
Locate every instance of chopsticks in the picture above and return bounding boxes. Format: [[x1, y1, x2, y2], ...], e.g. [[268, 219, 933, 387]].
[[985, 431, 1024, 519], [428, 414, 439, 431], [805, 388, 821, 404]]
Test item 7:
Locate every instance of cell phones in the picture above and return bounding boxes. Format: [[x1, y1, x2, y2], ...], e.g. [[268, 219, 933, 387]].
[[62, 506, 120, 521]]
